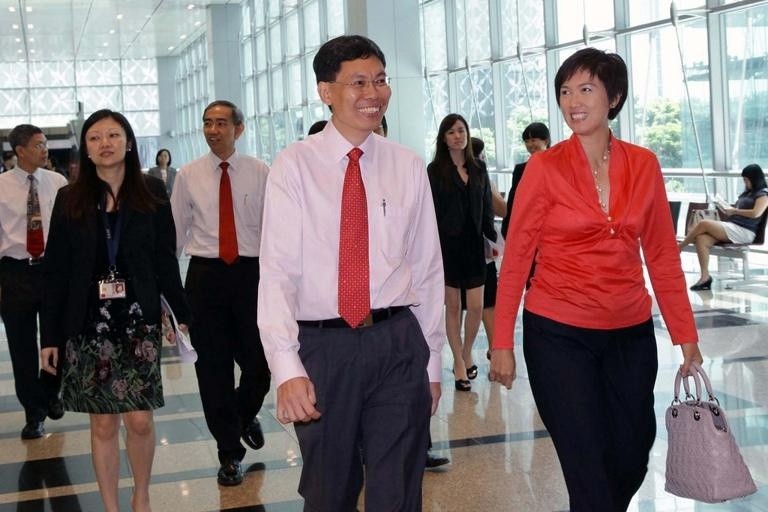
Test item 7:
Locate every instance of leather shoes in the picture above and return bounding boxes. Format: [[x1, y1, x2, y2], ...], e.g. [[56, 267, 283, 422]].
[[426, 452, 449, 468], [218, 460, 243, 486], [21, 423, 45, 438], [242, 416, 264, 448], [46, 398, 64, 419]]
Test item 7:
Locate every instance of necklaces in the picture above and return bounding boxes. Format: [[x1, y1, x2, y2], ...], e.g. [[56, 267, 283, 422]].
[[589, 144, 611, 216]]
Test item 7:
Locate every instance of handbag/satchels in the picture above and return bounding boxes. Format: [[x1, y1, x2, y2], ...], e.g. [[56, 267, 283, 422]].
[[687, 209, 721, 240], [666, 363, 756, 503]]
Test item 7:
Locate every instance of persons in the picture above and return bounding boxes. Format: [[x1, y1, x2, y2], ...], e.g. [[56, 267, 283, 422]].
[[306, 120, 326, 133], [426, 114, 496, 391], [489, 48, 702, 512], [170, 100, 271, 486], [678, 164, 768, 290], [374, 116, 451, 470], [0, 124, 68, 439], [46, 158, 63, 175], [501, 122, 551, 286], [460, 138, 507, 368], [0, 151, 18, 173], [148, 149, 177, 199], [38, 110, 190, 512], [257, 38, 446, 512]]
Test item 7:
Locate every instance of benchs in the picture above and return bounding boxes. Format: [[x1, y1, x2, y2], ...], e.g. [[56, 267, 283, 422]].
[[671, 202, 768, 280]]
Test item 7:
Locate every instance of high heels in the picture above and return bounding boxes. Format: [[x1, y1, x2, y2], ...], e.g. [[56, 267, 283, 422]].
[[690, 277, 712, 291]]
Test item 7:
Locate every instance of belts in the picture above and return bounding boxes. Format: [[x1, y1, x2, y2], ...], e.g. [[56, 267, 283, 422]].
[[297, 306, 406, 328], [21, 257, 43, 265]]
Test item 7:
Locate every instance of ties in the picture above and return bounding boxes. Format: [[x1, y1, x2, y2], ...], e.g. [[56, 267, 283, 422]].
[[338, 148, 370, 330], [26, 175, 45, 259], [219, 162, 238, 264]]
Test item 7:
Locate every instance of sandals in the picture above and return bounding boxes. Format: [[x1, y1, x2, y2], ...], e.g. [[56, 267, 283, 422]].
[[454, 380, 471, 391], [465, 364, 478, 380]]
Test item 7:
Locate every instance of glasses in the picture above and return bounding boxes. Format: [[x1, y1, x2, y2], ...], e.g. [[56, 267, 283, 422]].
[[330, 76, 391, 89]]
